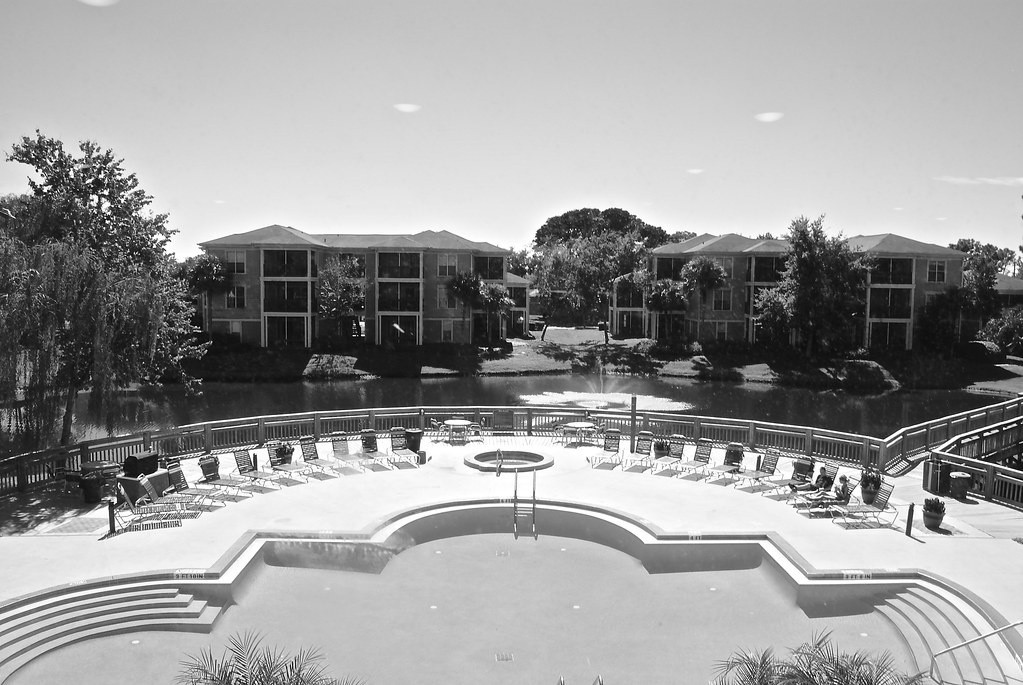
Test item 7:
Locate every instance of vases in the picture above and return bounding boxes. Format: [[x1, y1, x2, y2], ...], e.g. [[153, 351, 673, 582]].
[[860, 487, 878, 504]]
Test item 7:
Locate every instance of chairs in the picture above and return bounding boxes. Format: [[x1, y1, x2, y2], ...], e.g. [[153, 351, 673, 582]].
[[590, 428, 899, 527], [551, 419, 605, 448], [44, 462, 82, 496], [116, 426, 421, 529], [430, 417, 485, 446]]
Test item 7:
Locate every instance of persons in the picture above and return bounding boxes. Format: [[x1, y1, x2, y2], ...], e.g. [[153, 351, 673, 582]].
[[788, 467, 849, 500]]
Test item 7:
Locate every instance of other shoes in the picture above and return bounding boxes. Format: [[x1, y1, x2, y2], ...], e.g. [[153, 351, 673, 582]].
[[788, 483, 797, 492]]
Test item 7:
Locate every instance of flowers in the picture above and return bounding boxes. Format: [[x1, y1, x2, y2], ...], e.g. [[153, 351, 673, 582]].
[[860, 466, 882, 492]]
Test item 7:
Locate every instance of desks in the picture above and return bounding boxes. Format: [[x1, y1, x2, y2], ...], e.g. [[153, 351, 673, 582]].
[[567, 422, 595, 447], [443, 420, 471, 443], [80, 461, 120, 487]]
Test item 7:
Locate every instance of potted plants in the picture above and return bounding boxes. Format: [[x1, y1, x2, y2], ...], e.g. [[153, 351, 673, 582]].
[[276, 442, 295, 464], [923, 498, 945, 529], [653, 436, 669, 459]]
